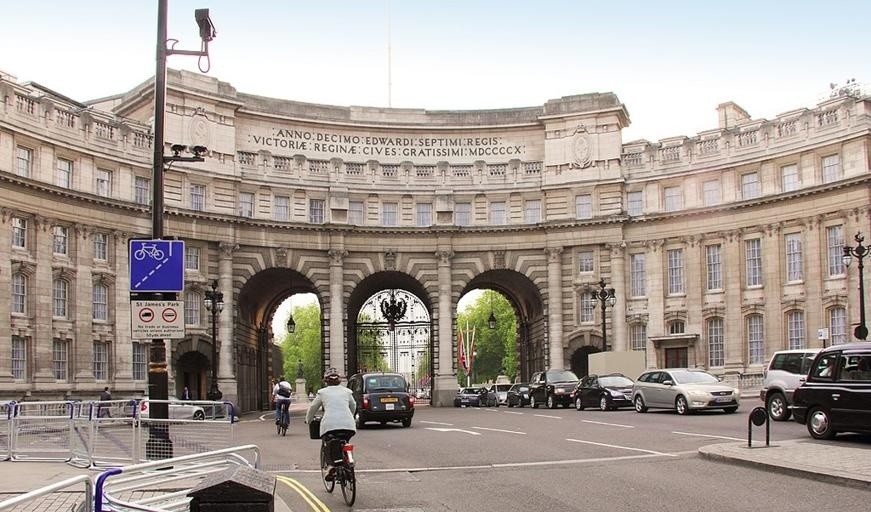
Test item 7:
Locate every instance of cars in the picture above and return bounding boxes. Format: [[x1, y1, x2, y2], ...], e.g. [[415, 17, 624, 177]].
[[123, 396, 206, 426], [507, 383, 531, 409], [414, 387, 432, 400], [633, 368, 740, 415], [454, 387, 488, 407], [488, 384, 514, 408], [573, 373, 634, 409]]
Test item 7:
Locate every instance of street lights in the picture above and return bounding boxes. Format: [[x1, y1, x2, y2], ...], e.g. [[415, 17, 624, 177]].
[[841, 232, 871, 339], [588, 277, 618, 352], [205, 280, 227, 401]]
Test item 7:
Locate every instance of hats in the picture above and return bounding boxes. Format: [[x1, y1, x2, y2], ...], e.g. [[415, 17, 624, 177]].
[[324, 367, 340, 377]]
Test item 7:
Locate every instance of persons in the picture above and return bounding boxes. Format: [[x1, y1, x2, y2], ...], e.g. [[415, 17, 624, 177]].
[[101, 386, 112, 419], [296, 359, 305, 378], [307, 365, 358, 480], [273, 373, 291, 425], [181, 385, 192, 406]]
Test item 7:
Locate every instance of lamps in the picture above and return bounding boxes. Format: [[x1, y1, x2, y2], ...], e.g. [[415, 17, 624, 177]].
[[287, 303, 296, 333], [488, 290, 497, 329]]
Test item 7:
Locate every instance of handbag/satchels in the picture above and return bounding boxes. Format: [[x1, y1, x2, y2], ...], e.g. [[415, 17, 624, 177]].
[[277, 385, 292, 398]]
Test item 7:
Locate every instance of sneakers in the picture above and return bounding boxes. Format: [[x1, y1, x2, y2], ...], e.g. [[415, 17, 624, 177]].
[[275, 419, 280, 425], [283, 424, 288, 428], [325, 466, 335, 482]]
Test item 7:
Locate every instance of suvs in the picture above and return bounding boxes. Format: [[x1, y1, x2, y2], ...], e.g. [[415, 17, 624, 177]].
[[759, 347, 828, 421], [346, 371, 415, 428], [529, 370, 581, 408], [793, 341, 871, 438]]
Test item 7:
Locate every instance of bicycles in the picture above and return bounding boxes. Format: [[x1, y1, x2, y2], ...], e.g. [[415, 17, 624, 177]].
[[274, 401, 290, 436], [303, 413, 357, 508]]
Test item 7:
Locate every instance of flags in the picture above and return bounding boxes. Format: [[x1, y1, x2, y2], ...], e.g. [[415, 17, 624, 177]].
[[471, 327, 478, 374], [458, 328, 466, 369]]
